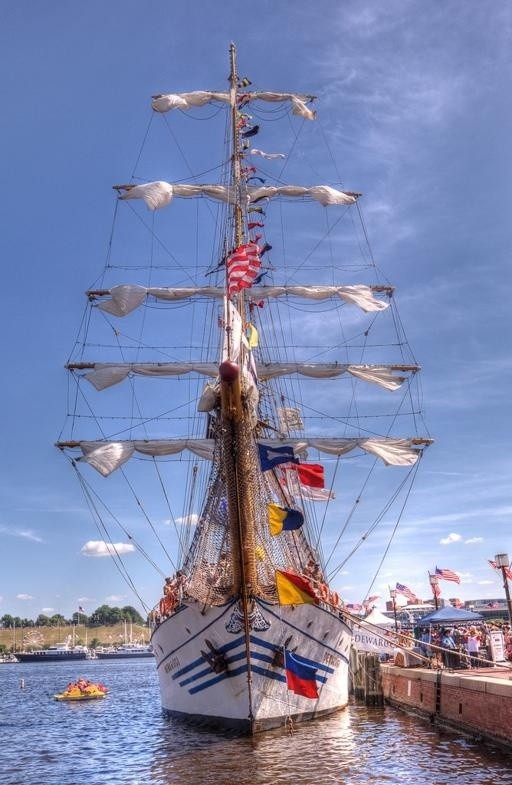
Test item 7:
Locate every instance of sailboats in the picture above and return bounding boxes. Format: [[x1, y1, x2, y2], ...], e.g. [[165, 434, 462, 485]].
[[146, 712, 360, 785], [46, 38, 436, 739]]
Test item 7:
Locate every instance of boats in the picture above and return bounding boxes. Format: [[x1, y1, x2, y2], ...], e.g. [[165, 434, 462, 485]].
[[53, 684, 110, 706], [13, 634, 88, 664], [95, 619, 154, 657]]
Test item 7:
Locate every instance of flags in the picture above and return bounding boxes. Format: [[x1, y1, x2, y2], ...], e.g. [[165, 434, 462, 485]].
[[347, 604, 362, 611], [228, 76, 271, 313], [395, 583, 415, 602], [265, 502, 304, 536], [435, 568, 460, 585], [430, 575, 440, 597], [489, 560, 512, 581], [276, 461, 326, 489], [285, 650, 320, 699], [257, 442, 294, 472], [275, 569, 320, 606], [363, 596, 379, 609]]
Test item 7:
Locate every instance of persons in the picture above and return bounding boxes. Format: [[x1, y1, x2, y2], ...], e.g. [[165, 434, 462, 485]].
[[162, 553, 230, 611], [302, 560, 330, 589], [385, 623, 512, 668]]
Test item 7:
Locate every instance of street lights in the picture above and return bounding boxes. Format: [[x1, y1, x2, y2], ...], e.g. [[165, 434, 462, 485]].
[[493, 552, 511, 630], [388, 588, 401, 636], [425, 569, 443, 639]]
